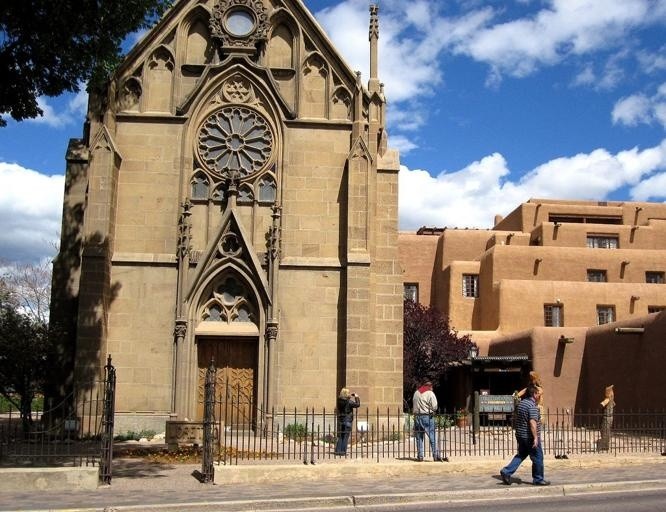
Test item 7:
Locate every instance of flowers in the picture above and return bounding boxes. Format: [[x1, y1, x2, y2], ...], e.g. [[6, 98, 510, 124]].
[[455, 408, 468, 415]]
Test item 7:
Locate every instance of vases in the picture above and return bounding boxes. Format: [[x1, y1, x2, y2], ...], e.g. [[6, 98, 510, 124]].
[[457, 416, 467, 429]]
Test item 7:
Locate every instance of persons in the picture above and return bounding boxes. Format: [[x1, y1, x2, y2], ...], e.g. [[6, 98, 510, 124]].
[[518, 370, 544, 425], [412, 376, 448, 462], [334, 387, 360, 456], [499, 384, 551, 486]]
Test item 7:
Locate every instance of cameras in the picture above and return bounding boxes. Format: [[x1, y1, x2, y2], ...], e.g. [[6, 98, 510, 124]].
[[351, 394, 355, 396]]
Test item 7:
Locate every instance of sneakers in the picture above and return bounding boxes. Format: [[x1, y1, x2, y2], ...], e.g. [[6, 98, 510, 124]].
[[500, 471, 511, 485], [533, 480, 550, 485]]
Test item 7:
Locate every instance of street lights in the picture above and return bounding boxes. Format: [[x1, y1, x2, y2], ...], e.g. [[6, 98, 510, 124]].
[[467, 344, 478, 445]]
